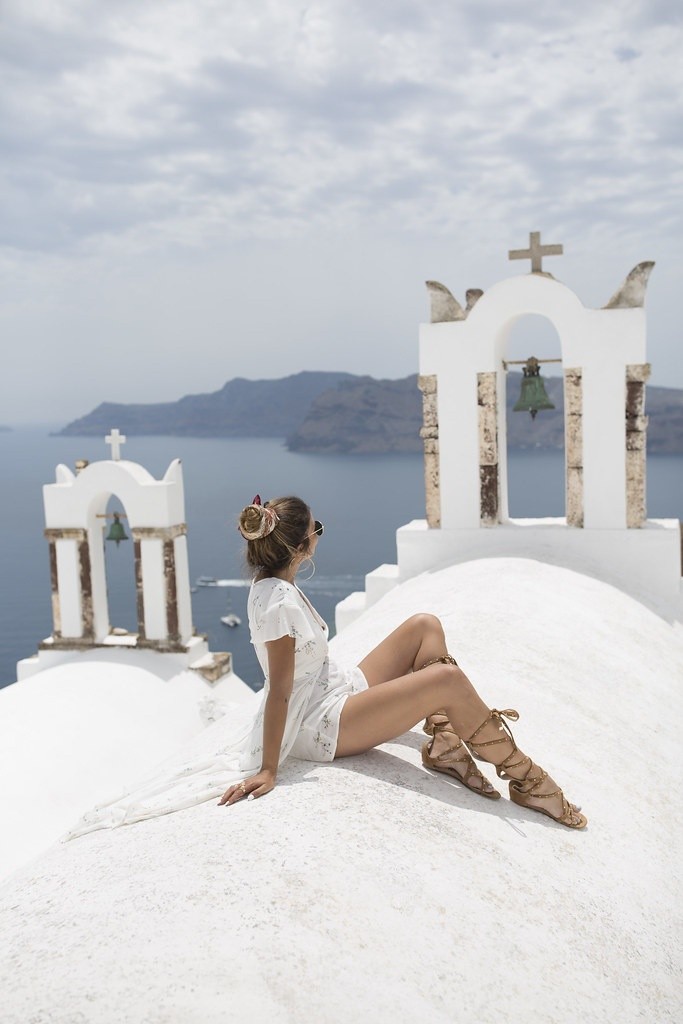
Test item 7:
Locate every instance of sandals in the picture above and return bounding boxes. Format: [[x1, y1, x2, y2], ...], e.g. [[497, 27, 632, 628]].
[[463, 708, 588, 828], [412, 654, 501, 799]]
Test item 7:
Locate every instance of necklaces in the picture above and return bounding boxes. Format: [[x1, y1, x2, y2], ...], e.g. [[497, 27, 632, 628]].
[[303, 598, 325, 630]]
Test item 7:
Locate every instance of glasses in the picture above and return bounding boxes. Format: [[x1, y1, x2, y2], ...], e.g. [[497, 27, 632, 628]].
[[303, 521, 324, 541]]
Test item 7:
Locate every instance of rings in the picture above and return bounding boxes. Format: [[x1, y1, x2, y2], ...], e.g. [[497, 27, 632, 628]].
[[235, 781, 246, 794]]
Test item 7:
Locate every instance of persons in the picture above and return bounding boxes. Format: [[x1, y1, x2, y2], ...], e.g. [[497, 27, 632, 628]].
[[217, 494, 587, 845]]
